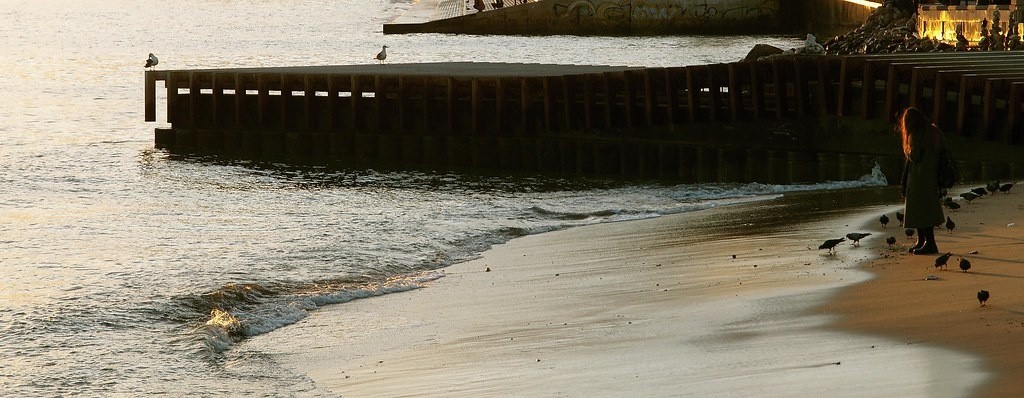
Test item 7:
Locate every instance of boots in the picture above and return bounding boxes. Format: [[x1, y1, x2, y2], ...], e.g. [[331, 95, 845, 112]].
[[908, 234, 938, 254]]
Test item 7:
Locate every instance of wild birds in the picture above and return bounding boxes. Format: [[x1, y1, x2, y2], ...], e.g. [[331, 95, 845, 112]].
[[819, 237, 846, 252], [896, 212, 904, 225], [846, 233, 873, 244], [977, 289, 990, 307], [934, 252, 953, 271], [999, 184, 1013, 194], [887, 237, 897, 246], [879, 214, 889, 228], [959, 187, 987, 203], [946, 216, 955, 233], [905, 227, 914, 239], [987, 181, 1000, 196], [374, 44, 391, 66], [144, 53, 159, 71], [959, 257, 971, 273], [942, 196, 960, 211]]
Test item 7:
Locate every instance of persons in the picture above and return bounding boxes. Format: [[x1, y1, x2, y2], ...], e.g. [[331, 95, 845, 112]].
[[473, 0, 485, 13], [900, 107, 946, 256], [491, 0, 505, 9]]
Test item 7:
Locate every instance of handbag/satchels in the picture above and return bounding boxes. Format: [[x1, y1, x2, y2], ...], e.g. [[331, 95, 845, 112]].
[[938, 128, 957, 189]]
